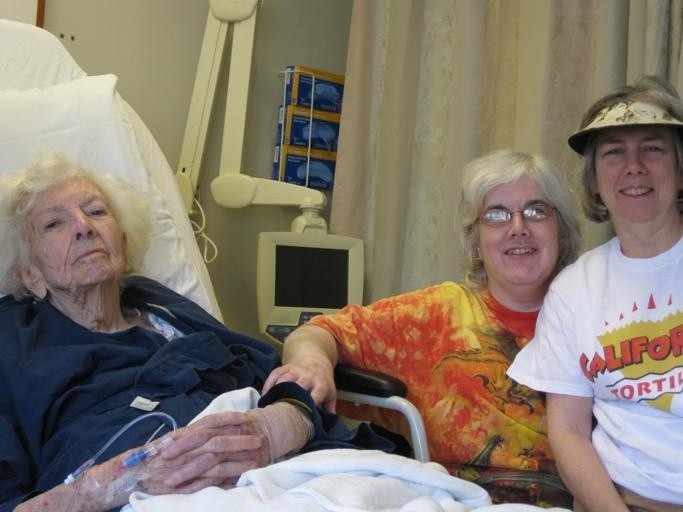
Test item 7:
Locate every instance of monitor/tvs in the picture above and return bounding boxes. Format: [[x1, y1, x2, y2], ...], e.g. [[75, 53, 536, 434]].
[[256, 231, 365, 351]]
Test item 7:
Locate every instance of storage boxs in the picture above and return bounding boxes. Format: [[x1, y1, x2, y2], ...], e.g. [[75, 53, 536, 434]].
[[271, 65, 345, 192]]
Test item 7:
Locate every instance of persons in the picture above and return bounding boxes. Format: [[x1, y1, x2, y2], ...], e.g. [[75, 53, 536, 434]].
[[503, 71, 681, 511], [258, 147, 586, 511], [0, 146, 414, 511]]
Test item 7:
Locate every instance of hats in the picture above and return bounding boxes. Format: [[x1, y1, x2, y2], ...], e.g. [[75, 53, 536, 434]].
[[567, 97, 683, 154]]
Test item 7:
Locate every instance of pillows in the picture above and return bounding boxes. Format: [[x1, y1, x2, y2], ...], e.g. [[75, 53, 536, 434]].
[[0, 75, 197, 306]]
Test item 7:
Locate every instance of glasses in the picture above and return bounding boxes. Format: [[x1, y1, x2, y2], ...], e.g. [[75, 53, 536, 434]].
[[477, 203, 559, 228]]
